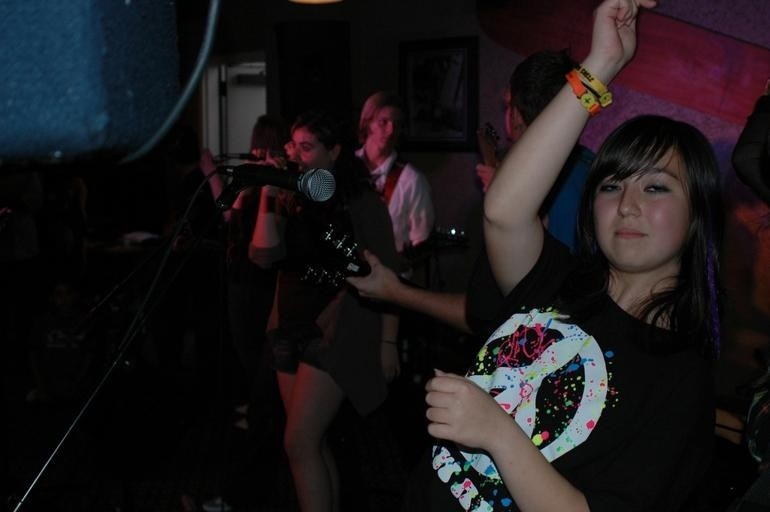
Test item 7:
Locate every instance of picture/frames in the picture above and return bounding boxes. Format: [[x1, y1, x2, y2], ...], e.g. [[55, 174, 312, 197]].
[[397, 35, 479, 154]]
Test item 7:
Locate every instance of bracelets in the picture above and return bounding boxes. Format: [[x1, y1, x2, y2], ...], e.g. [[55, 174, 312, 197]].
[[565, 64, 613, 116]]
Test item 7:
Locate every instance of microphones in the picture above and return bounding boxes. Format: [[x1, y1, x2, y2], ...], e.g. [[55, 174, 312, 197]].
[[221, 150, 288, 161], [215, 164, 335, 202]]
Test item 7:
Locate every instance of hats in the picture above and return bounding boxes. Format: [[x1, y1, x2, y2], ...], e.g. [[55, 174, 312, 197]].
[[510, 48, 580, 128]]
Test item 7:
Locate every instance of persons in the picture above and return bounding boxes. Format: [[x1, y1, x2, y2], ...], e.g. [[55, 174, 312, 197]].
[[367, 1, 726, 512], [730, 77, 769, 211], [247, 117, 397, 512], [732, 368, 770, 512], [352, 91, 436, 253], [197, 116, 288, 431], [346, 50, 597, 336]]
